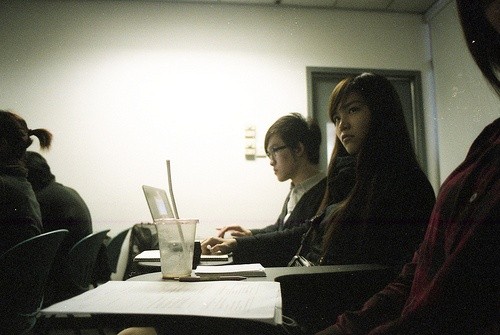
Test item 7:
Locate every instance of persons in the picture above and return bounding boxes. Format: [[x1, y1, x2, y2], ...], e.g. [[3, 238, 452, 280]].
[[197, 73, 438, 335], [202, 115, 328, 255], [0, 111, 53, 335], [323, 1, 500, 335], [22, 152, 90, 294]]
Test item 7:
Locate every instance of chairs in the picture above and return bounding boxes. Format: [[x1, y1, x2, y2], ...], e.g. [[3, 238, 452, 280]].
[[1, 229, 67, 334], [68, 229, 110, 289], [107, 227, 131, 274]]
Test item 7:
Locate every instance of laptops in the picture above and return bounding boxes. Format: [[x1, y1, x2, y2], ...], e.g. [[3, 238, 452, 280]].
[[142, 185, 228, 261]]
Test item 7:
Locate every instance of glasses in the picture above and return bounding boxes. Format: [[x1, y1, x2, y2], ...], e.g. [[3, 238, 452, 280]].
[[266, 143, 288, 158]]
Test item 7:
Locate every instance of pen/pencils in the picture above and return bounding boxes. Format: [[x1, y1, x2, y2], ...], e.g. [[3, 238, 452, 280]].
[[180, 276, 248, 282]]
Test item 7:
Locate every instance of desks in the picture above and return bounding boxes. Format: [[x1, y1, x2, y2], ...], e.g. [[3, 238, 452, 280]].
[[41, 243, 381, 325]]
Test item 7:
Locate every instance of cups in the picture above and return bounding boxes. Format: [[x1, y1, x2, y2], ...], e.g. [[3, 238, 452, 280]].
[[155, 219, 198, 278], [168, 240, 201, 270]]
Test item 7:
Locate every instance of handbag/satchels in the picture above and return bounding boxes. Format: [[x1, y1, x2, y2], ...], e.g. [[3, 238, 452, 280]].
[[95, 223, 202, 284]]
